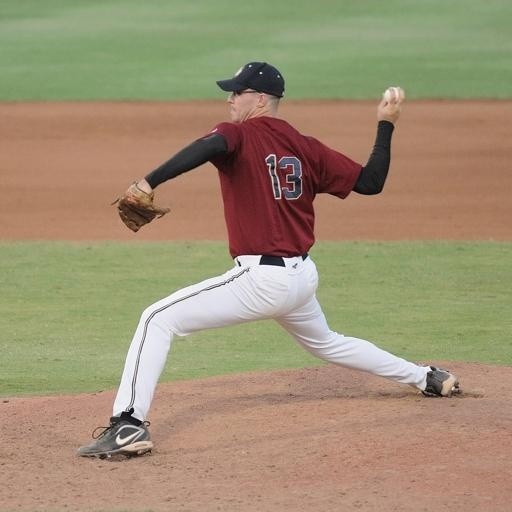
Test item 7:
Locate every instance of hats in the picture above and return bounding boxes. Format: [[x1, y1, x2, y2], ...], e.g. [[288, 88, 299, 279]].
[[217, 62, 286, 97]]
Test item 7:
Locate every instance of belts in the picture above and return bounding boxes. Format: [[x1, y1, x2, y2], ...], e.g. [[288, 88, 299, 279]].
[[235, 253, 308, 266]]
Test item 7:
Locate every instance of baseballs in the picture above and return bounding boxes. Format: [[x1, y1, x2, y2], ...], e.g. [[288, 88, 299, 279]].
[[384, 86, 400, 103]]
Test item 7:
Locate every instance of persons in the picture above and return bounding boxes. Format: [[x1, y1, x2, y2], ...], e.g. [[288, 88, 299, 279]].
[[66, 61, 459, 460]]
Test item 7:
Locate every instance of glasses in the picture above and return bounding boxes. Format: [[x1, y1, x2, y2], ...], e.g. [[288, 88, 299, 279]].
[[231, 88, 260, 95]]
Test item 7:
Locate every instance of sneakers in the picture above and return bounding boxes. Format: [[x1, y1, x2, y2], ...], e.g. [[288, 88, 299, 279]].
[[422, 366, 461, 396], [79, 408, 153, 459]]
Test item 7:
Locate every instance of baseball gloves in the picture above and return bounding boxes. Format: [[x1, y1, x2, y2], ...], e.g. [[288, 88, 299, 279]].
[[111, 180, 171, 232]]
